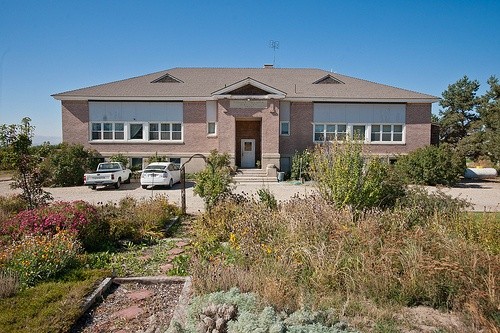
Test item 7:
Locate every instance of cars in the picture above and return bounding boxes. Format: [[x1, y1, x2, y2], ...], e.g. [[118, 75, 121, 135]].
[[140, 162, 181, 189]]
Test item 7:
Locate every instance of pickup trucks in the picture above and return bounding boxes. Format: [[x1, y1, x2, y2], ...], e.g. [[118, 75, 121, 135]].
[[82, 161, 133, 189]]
